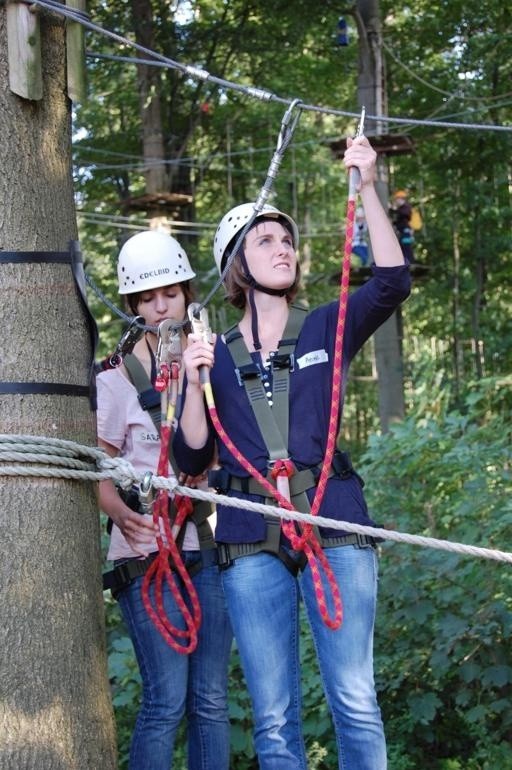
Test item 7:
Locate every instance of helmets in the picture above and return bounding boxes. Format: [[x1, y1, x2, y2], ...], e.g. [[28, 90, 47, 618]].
[[213, 202, 300, 290], [116, 229, 197, 295]]
